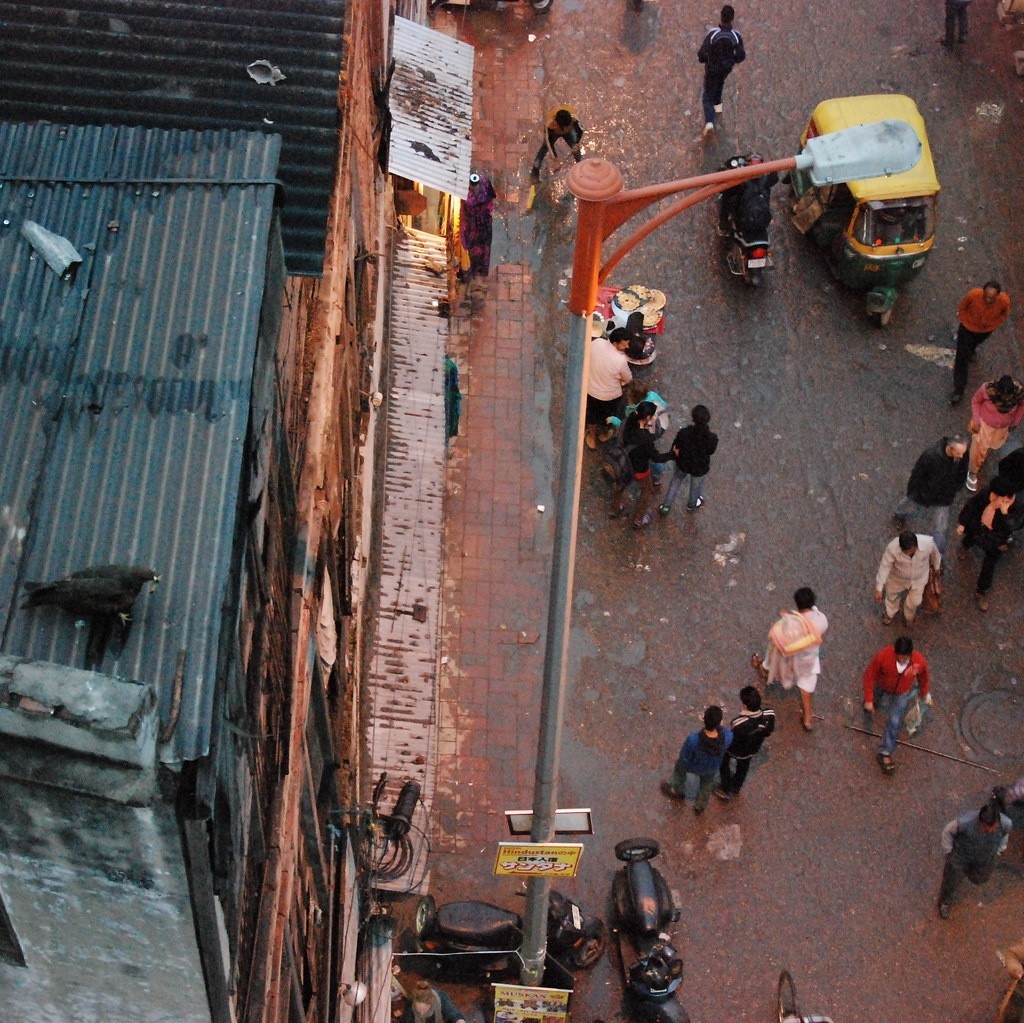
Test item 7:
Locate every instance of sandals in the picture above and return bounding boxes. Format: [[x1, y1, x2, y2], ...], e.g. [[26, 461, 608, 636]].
[[879, 753, 896, 774]]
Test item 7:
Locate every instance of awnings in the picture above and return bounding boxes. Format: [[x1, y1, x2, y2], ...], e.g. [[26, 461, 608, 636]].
[[386, 14, 475, 200]]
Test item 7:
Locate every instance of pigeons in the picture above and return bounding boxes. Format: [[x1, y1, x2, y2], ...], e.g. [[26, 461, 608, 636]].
[[19, 565, 159, 626]]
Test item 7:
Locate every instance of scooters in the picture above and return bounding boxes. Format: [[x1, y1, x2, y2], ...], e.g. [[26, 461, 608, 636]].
[[609, 837, 690, 1023], [408, 882, 606, 983], [717, 156, 775, 291]]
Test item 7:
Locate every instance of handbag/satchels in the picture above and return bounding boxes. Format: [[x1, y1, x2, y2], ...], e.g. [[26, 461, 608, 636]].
[[921, 565, 944, 617], [768, 610, 820, 656]]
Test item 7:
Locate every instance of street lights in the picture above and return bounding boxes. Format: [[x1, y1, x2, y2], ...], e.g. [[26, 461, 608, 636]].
[[515, 119, 928, 983]]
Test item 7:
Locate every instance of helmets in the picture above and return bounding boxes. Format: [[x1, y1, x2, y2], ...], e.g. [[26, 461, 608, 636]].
[[743, 156, 764, 167]]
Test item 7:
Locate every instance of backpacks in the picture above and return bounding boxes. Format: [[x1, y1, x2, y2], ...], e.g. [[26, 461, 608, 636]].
[[601, 430, 648, 490], [707, 26, 737, 75], [737, 175, 772, 231]]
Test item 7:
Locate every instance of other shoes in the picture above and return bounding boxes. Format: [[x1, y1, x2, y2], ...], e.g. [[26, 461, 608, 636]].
[[703, 122, 714, 136], [754, 652, 768, 678], [882, 613, 893, 625], [804, 722, 814, 729], [713, 784, 730, 800], [951, 390, 962, 404], [661, 781, 684, 799], [634, 515, 650, 529], [714, 103, 723, 113], [894, 515, 906, 530], [957, 546, 966, 560], [687, 495, 704, 512], [940, 907, 951, 919], [659, 504, 669, 514], [611, 503, 628, 518], [976, 591, 989, 610], [905, 620, 914, 629]]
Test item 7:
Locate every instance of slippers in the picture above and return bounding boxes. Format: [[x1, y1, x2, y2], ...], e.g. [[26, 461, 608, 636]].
[[966, 471, 978, 492]]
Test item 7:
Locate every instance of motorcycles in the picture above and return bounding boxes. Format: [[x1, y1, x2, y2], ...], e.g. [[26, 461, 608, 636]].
[[782, 94, 941, 330]]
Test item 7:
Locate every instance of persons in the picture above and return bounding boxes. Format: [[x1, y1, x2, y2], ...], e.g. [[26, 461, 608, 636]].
[[697, 5, 745, 138], [713, 687, 776, 802], [530, 105, 584, 178], [750, 587, 828, 732], [891, 376, 1024, 613], [717, 151, 779, 237], [951, 281, 1011, 402], [939, 0, 972, 47], [935, 778, 1024, 1023], [661, 707, 733, 815], [863, 637, 933, 773], [875, 530, 943, 628], [459, 173, 496, 280], [584, 326, 719, 529]]
